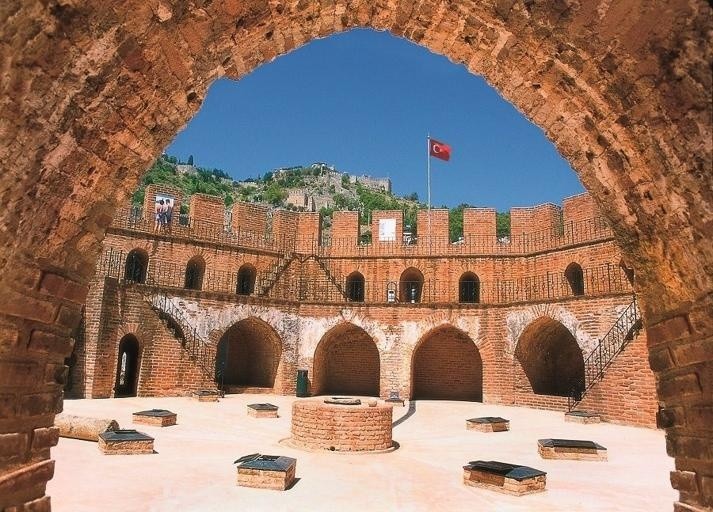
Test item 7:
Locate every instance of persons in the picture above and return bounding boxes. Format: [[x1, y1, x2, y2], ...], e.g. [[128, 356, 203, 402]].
[[162, 198, 172, 235], [153, 200, 165, 234]]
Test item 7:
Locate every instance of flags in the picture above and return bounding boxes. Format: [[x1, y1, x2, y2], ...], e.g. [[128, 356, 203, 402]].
[[429, 137, 451, 161]]
